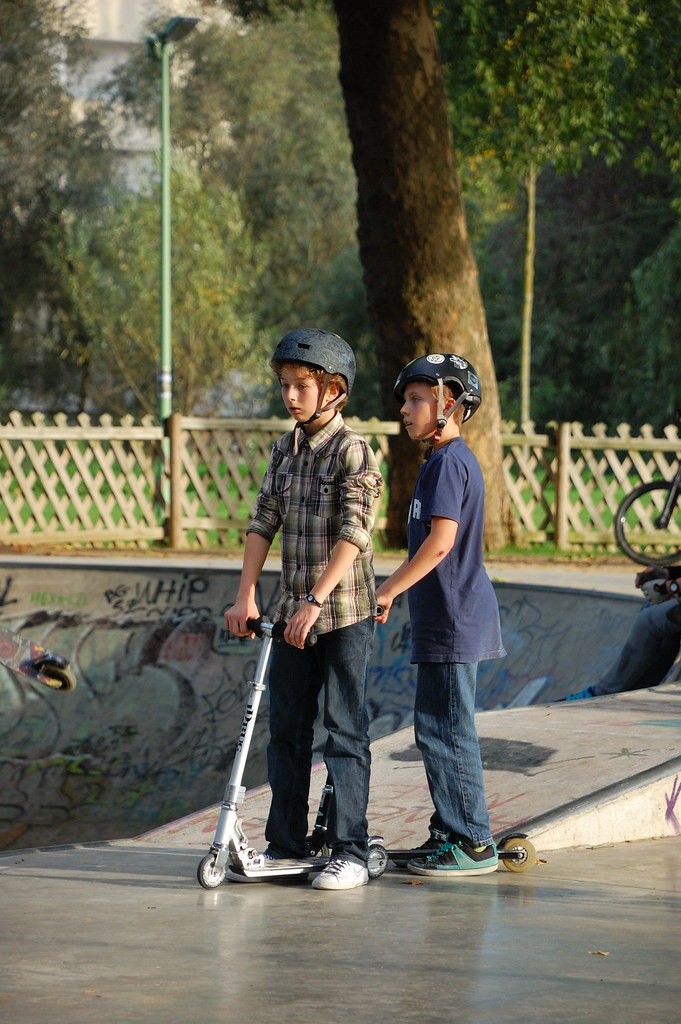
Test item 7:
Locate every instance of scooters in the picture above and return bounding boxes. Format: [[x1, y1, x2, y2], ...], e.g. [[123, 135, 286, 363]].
[[197, 616, 388, 889], [304, 606, 536, 873]]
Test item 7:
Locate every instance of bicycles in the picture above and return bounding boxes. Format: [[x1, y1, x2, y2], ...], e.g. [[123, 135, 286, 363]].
[[615, 465, 681, 567]]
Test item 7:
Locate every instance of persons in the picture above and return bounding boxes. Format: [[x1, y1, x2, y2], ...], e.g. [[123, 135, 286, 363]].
[[375, 354, 507, 876], [566, 561, 681, 701], [225, 330, 382, 890]]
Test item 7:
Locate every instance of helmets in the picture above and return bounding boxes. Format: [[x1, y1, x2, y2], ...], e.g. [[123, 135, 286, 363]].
[[394, 354, 481, 424], [272, 328, 355, 394]]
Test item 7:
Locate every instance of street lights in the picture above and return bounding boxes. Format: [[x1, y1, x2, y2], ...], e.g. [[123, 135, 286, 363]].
[[149, 17, 206, 431]]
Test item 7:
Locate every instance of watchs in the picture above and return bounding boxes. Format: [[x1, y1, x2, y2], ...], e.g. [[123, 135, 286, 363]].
[[306, 594, 324, 608], [669, 578, 679, 597]]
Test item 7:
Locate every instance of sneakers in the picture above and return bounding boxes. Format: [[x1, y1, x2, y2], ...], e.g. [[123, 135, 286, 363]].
[[312, 859, 369, 890], [408, 833, 498, 877], [393, 827, 451, 867], [224, 854, 308, 882]]
